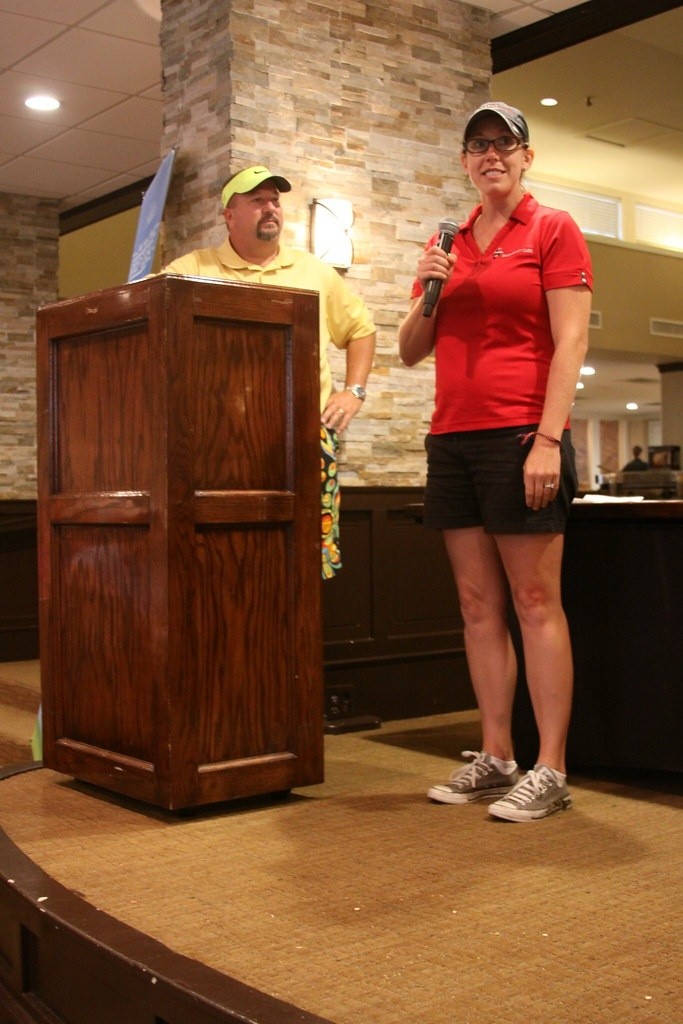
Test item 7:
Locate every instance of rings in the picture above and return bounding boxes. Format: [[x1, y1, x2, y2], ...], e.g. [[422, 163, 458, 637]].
[[339, 409, 345, 415], [543, 484, 554, 488]]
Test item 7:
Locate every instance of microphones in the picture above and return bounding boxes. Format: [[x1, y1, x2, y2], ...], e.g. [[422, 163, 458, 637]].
[[422, 217, 460, 316]]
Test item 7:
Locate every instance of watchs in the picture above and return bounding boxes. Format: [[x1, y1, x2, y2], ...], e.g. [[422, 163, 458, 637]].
[[345, 384, 366, 400]]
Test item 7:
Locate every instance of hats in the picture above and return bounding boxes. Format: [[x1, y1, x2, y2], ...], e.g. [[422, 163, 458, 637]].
[[221, 166, 291, 209], [462, 101, 529, 149]]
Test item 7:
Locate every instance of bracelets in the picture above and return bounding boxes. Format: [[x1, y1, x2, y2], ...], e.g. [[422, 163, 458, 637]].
[[516, 431, 562, 446]]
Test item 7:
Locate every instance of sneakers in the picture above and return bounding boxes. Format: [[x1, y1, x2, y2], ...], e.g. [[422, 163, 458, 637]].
[[427, 750, 521, 803], [464, 740, 572, 822]]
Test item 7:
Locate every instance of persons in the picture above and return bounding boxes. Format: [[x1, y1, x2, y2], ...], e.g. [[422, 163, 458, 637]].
[[396, 102, 595, 823], [621, 446, 648, 471], [130, 164, 378, 581]]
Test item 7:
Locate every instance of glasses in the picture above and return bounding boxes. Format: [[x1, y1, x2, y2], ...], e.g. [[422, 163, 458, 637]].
[[462, 136, 526, 154]]
